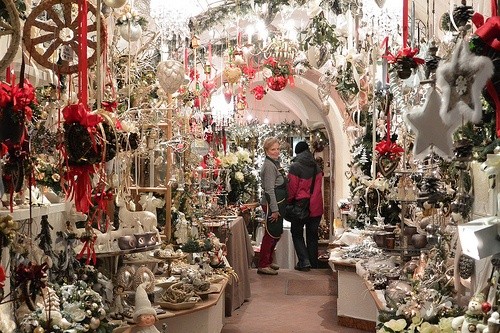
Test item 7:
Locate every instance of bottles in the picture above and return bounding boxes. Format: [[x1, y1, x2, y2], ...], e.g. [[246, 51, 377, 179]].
[[334, 217, 342, 231]]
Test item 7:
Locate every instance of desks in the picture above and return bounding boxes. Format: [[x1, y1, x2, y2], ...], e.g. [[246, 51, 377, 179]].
[[201, 216, 255, 316], [257, 220, 306, 269]]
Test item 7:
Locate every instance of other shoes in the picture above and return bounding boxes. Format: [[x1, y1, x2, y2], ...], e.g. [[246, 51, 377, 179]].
[[257, 268, 278, 275], [309, 260, 316, 269], [270, 263, 279, 270], [294, 260, 310, 271]]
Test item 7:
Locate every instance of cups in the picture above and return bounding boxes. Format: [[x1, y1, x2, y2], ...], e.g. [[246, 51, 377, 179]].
[[386, 238, 395, 249]]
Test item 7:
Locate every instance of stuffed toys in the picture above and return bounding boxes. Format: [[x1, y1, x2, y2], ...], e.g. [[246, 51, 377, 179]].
[[452, 295, 483, 333]]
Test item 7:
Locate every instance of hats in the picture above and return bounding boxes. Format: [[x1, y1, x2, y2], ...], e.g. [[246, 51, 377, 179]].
[[295, 142, 309, 154]]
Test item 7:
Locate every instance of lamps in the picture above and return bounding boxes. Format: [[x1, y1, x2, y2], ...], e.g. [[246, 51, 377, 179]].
[[457, 216, 500, 260]]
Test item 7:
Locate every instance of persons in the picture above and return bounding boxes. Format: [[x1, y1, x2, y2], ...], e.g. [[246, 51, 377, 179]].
[[257, 138, 286, 275], [132, 308, 160, 333], [287, 142, 324, 270]]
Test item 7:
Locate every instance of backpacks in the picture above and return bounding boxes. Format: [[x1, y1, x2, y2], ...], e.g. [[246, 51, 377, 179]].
[[284, 197, 309, 222]]
